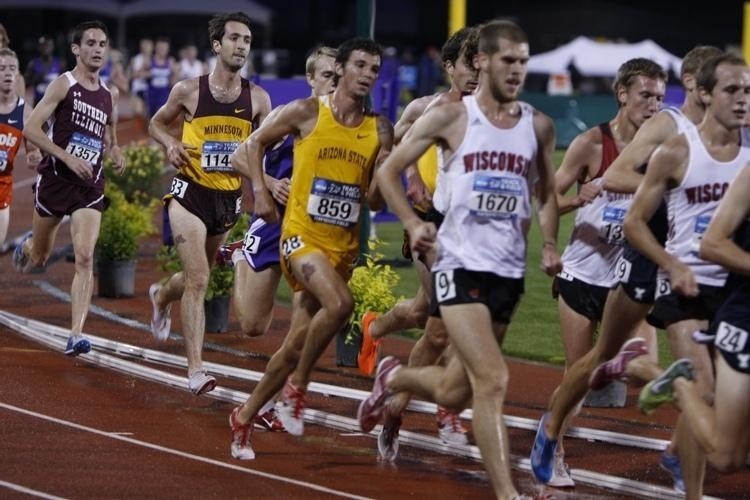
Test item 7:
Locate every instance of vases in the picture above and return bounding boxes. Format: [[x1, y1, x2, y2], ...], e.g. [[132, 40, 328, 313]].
[[583, 377, 626, 408]]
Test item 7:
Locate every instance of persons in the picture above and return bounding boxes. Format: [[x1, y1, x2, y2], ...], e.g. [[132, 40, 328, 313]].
[[229, 37, 395, 459], [0, 25, 126, 356], [148, 10, 271, 395], [532, 46, 749, 500], [97, 37, 205, 144], [357, 22, 563, 500]]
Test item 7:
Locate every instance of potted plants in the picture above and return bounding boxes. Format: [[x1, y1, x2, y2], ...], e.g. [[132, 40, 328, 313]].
[[333, 239, 399, 367], [156, 209, 250, 332], [98, 183, 157, 299]]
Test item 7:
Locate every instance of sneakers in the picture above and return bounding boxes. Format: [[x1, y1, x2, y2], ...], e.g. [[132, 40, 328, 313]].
[[640, 359, 694, 416], [231, 405, 256, 461], [65, 334, 91, 357], [546, 452, 575, 486], [214, 240, 244, 268], [658, 452, 687, 496], [357, 356, 402, 434], [275, 381, 306, 437], [377, 408, 400, 461], [188, 369, 217, 398], [529, 412, 559, 484], [435, 402, 467, 446], [148, 282, 173, 345], [589, 337, 648, 392], [357, 311, 381, 375], [12, 231, 33, 273], [252, 407, 288, 432], [511, 488, 556, 500]]
